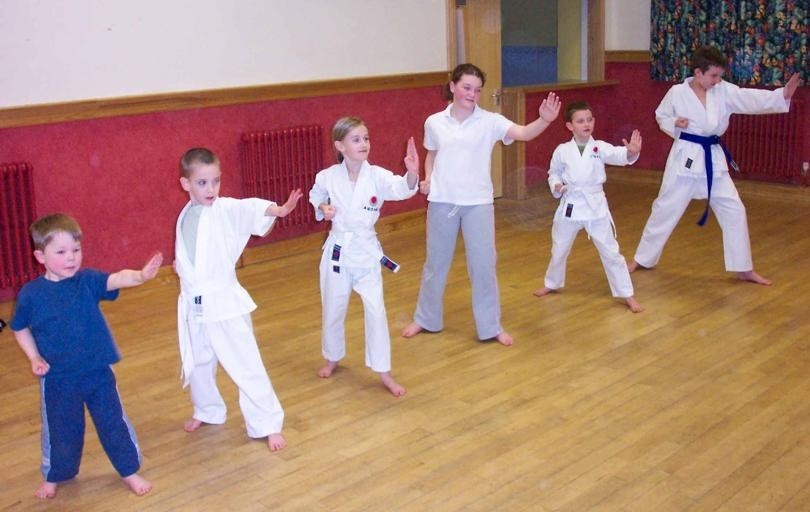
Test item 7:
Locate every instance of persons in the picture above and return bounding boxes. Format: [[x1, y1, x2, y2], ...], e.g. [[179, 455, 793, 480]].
[[9, 213, 164, 500], [532, 101, 642, 313], [401, 62, 562, 346], [309, 117, 420, 398], [173, 147, 304, 452], [628, 44, 800, 285]]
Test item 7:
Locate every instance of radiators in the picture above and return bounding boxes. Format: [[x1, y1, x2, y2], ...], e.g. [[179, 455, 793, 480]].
[[242, 126, 333, 229], [0, 162, 39, 288]]
